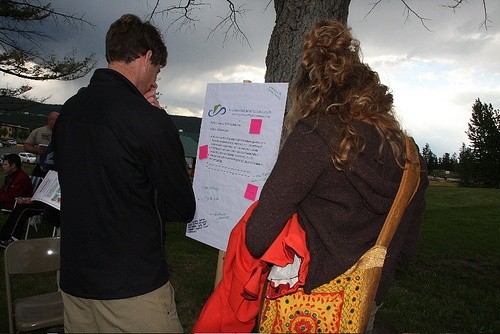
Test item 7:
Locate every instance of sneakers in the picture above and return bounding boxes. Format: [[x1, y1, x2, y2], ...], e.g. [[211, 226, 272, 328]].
[[0, 236, 18, 247]]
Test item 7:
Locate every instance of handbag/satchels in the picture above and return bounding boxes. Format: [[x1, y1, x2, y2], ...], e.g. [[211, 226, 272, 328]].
[[257, 246, 387, 334]]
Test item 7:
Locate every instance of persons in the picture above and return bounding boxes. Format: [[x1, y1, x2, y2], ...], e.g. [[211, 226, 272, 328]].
[[245, 19, 435, 334], [52, 13, 197, 334], [0, 112, 61, 248]]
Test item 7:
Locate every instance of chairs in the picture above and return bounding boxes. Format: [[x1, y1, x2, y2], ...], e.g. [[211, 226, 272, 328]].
[[4, 212, 66, 334]]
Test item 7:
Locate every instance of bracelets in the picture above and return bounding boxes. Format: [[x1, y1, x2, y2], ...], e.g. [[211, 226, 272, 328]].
[[35, 147, 38, 153]]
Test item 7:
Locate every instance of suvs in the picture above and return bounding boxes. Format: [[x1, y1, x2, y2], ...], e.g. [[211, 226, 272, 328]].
[[19, 152, 36, 163], [8, 140, 17, 146]]
